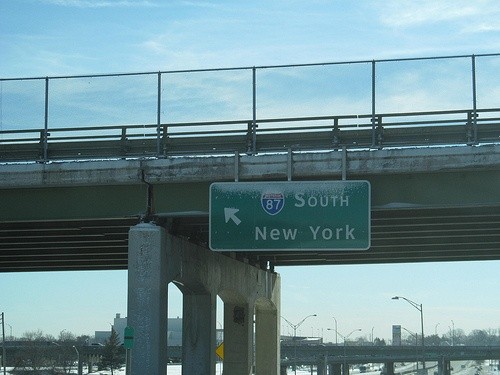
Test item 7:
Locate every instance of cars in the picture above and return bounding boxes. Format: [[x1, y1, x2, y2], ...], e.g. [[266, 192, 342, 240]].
[[91, 342, 105, 347]]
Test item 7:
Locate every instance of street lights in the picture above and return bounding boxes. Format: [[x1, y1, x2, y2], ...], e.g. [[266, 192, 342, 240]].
[[281, 313, 318, 375], [72, 345, 80, 375], [391, 296, 426, 369], [327, 328, 362, 375], [395, 327, 418, 370]]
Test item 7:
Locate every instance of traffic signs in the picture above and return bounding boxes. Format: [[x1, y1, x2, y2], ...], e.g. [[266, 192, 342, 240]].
[[208, 181, 371, 252]]
